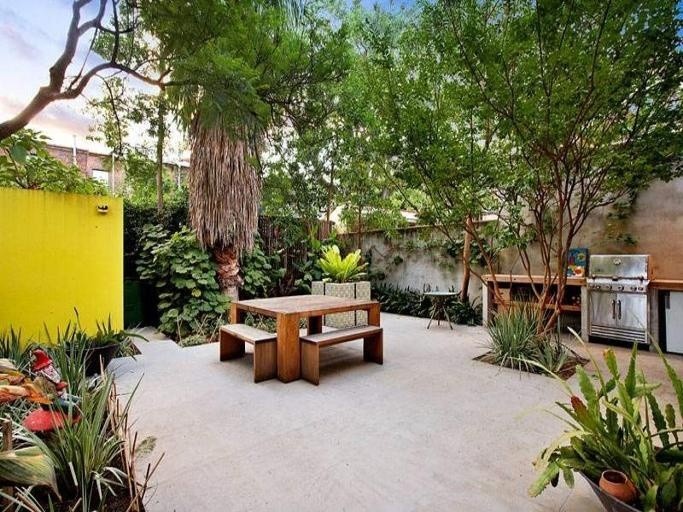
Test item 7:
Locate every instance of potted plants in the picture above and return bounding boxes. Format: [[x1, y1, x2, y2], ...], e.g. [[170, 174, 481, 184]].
[[513, 325, 682, 512], [53, 319, 148, 378], [310, 243, 371, 327]]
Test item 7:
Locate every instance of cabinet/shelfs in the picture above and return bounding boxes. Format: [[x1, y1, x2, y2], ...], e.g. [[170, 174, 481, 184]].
[[480, 274, 587, 346], [587, 290, 648, 343]]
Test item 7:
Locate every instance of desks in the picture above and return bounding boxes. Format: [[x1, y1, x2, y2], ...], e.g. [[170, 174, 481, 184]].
[[228, 292, 381, 383], [423, 291, 458, 330]]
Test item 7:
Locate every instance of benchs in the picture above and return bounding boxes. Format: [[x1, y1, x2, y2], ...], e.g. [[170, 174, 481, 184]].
[[218, 322, 384, 387]]
